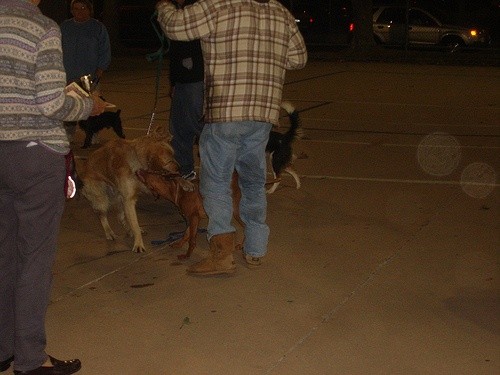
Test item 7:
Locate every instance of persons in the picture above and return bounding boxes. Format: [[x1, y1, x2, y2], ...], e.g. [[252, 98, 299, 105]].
[[167, 0, 206, 181], [0, 0, 109, 375], [155, 0, 308, 276], [58, 0, 111, 149]]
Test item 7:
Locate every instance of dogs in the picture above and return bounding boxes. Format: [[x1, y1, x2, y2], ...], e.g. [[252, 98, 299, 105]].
[[75, 124, 177, 253], [78, 109, 126, 149], [136, 168, 246, 261], [265, 101, 303, 195]]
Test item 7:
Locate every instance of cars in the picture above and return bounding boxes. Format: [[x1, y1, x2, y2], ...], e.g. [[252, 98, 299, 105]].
[[282, 1, 374, 49], [371, 2, 489, 59]]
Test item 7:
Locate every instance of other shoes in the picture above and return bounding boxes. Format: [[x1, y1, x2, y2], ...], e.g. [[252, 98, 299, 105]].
[[181, 171, 196, 181]]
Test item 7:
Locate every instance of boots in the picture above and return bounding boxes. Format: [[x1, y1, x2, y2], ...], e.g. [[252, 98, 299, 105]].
[[244, 253, 264, 269], [186, 232, 236, 276]]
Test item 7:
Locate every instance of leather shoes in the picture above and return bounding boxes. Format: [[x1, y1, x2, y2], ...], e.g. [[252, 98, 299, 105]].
[[0, 354, 15, 371], [13, 354, 81, 375]]
